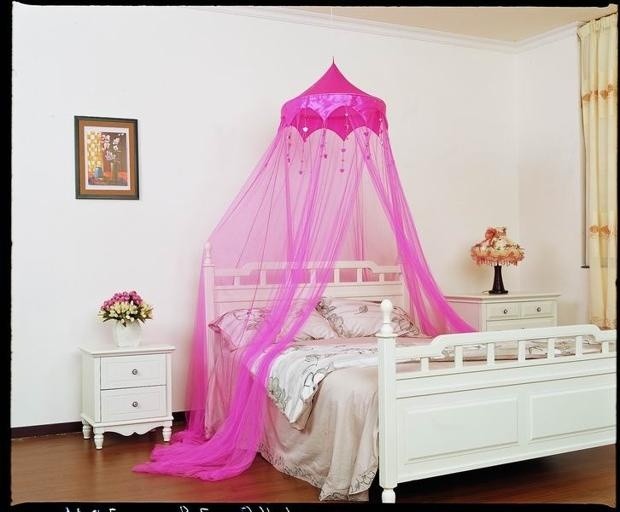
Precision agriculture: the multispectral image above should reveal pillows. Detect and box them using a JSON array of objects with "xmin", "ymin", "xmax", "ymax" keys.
[
  {"xmin": 314, "ymin": 294, "xmax": 424, "ymax": 338},
  {"xmin": 206, "ymin": 299, "xmax": 339, "ymax": 354}
]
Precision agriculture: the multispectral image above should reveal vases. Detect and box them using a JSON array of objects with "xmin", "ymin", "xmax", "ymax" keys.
[
  {"xmin": 484, "ymin": 264, "xmax": 513, "ymax": 297},
  {"xmin": 111, "ymin": 316, "xmax": 147, "ymax": 349}
]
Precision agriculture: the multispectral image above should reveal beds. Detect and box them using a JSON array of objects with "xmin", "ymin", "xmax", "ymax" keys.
[{"xmin": 201, "ymin": 250, "xmax": 620, "ymax": 510}]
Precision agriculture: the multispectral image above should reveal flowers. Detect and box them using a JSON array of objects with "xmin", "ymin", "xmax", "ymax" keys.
[
  {"xmin": 95, "ymin": 288, "xmax": 156, "ymax": 329},
  {"xmin": 468, "ymin": 223, "xmax": 527, "ymax": 270}
]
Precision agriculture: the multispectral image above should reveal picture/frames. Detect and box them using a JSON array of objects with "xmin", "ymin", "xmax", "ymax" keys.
[{"xmin": 72, "ymin": 113, "xmax": 144, "ymax": 204}]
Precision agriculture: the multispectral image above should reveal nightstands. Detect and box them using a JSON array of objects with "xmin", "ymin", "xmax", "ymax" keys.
[
  {"xmin": 78, "ymin": 340, "xmax": 177, "ymax": 452},
  {"xmin": 437, "ymin": 289, "xmax": 563, "ymax": 334}
]
